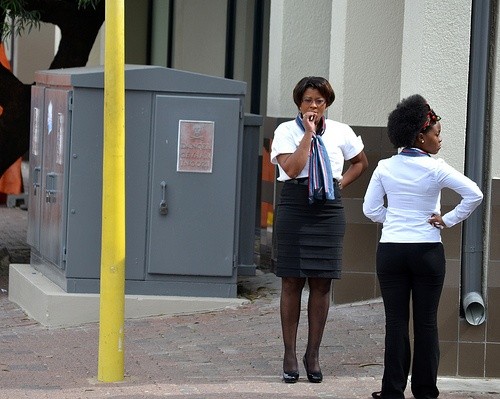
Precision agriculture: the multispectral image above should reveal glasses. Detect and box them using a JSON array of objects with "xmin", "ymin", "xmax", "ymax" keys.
[{"xmin": 303, "ymin": 97, "xmax": 326, "ymax": 106}]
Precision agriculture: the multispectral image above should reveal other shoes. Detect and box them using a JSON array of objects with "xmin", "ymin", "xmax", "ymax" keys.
[{"xmin": 371, "ymin": 391, "xmax": 383, "ymax": 399}]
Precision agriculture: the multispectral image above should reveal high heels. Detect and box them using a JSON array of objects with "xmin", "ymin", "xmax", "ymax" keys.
[
  {"xmin": 283, "ymin": 357, "xmax": 299, "ymax": 383},
  {"xmin": 303, "ymin": 353, "xmax": 322, "ymax": 382}
]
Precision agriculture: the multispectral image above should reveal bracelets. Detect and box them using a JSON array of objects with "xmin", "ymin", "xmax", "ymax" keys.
[{"xmin": 338, "ymin": 182, "xmax": 342, "ymax": 189}]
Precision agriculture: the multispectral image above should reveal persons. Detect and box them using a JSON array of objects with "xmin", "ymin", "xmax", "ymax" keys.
[
  {"xmin": 363, "ymin": 95, "xmax": 484, "ymax": 399},
  {"xmin": 271, "ymin": 76, "xmax": 369, "ymax": 382}
]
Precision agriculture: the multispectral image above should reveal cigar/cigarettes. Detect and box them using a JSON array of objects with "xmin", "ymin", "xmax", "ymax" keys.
[{"xmin": 310, "ymin": 116, "xmax": 313, "ymax": 120}]
